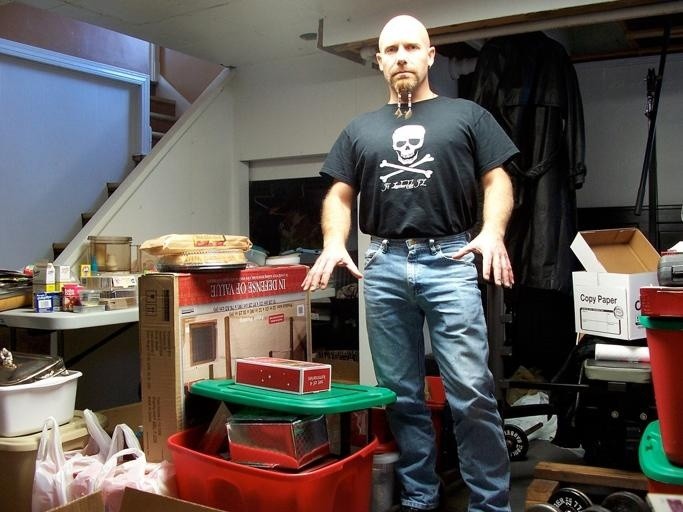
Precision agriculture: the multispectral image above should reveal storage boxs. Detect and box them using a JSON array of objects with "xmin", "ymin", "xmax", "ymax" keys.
[{"xmin": 559, "ymin": 223, "xmax": 682, "ymax": 511}]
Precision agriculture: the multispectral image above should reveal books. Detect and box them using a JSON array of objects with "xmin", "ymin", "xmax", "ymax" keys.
[{"xmin": 264, "ymin": 252, "xmax": 319, "ymax": 266}]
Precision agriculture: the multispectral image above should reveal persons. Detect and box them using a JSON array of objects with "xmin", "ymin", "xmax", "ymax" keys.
[{"xmin": 299, "ymin": 14, "xmax": 521, "ymax": 511}]
